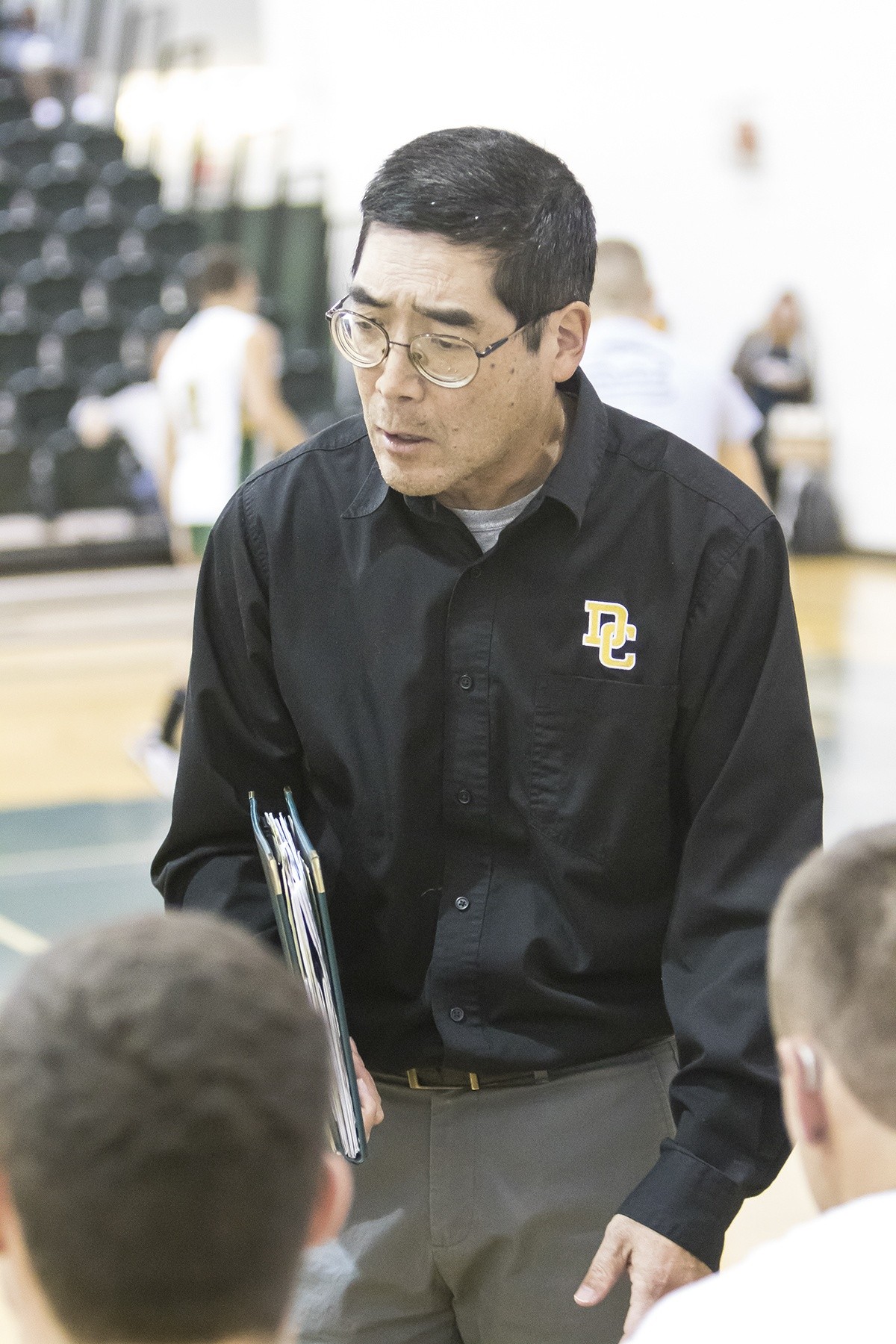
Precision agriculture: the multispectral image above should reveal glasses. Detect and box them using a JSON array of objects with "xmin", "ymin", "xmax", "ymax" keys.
[{"xmin": 324, "ymin": 286, "xmax": 551, "ymax": 389}]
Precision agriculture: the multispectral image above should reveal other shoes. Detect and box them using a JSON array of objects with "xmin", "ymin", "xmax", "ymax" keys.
[{"xmin": 139, "ymin": 737, "xmax": 180, "ymax": 793}]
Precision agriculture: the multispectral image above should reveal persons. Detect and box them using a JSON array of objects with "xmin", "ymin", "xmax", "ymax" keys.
[
  {"xmin": 31, "ymin": 240, "xmax": 312, "ymax": 565},
  {"xmin": 579, "ymin": 240, "xmax": 770, "ymax": 510},
  {"xmin": 732, "ymin": 290, "xmax": 834, "ymax": 552},
  {"xmin": 148, "ymin": 125, "xmax": 824, "ymax": 1344},
  {"xmin": 623, "ymin": 820, "xmax": 896, "ymax": 1344},
  {"xmin": 0, "ymin": 910, "xmax": 354, "ymax": 1344}
]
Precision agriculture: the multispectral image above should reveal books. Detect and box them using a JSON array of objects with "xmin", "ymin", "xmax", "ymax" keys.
[{"xmin": 246, "ymin": 786, "xmax": 367, "ymax": 1163}]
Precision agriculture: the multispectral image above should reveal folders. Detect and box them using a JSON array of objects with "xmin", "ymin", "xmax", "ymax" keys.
[{"xmin": 246, "ymin": 785, "xmax": 366, "ymax": 1164}]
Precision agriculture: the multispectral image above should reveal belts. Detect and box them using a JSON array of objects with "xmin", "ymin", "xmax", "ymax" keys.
[{"xmin": 366, "ymin": 1059, "xmax": 558, "ymax": 1090}]
